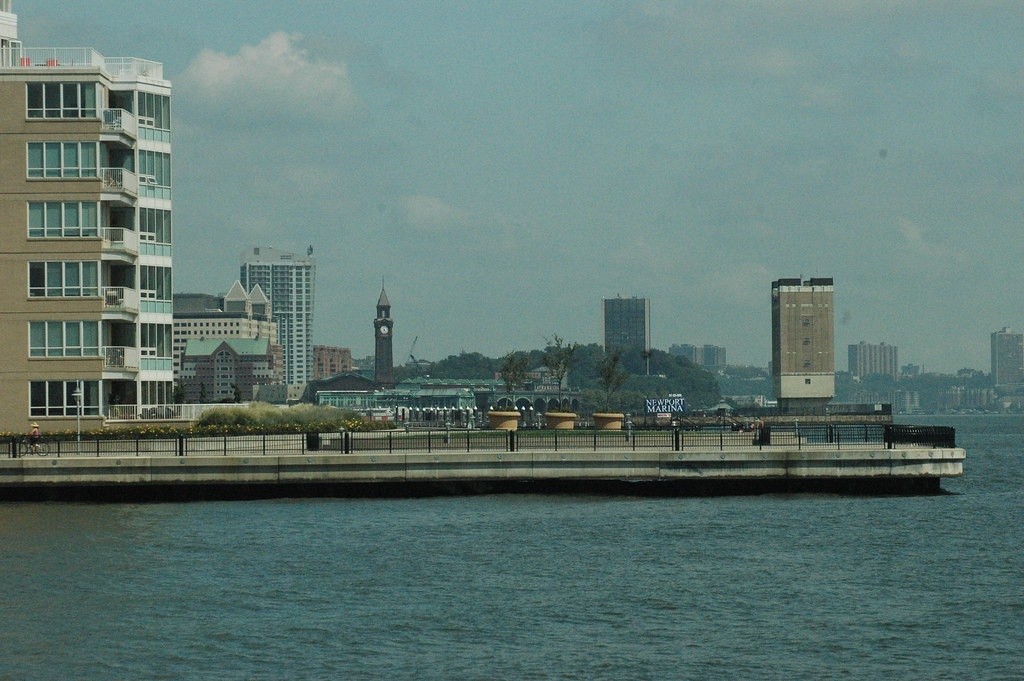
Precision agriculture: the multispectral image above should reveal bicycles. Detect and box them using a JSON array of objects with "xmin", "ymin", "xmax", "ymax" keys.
[{"xmin": 15, "ymin": 436, "xmax": 50, "ymax": 456}]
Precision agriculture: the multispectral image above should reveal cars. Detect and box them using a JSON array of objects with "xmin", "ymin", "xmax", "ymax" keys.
[{"xmin": 141, "ymin": 406, "xmax": 174, "ymax": 419}]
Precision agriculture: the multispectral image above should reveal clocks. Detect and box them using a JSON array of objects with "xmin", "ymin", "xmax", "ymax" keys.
[{"xmin": 380, "ymin": 325, "xmax": 389, "ymax": 334}]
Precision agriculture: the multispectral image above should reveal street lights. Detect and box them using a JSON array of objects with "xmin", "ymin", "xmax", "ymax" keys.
[
  {"xmin": 396, "ymin": 405, "xmax": 478, "ymax": 427},
  {"xmin": 522, "ymin": 406, "xmax": 534, "ymax": 426},
  {"xmin": 72, "ymin": 388, "xmax": 83, "ymax": 453}
]
